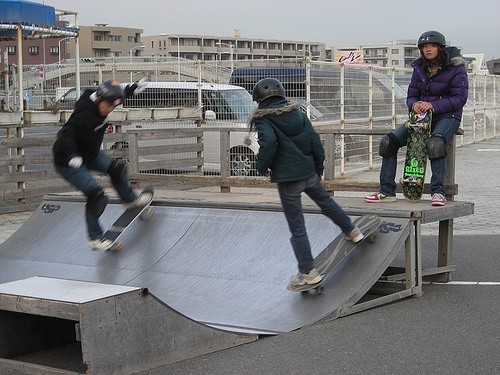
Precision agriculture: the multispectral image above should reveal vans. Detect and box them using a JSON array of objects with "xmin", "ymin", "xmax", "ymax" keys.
[
  {"xmin": 100, "ymin": 81, "xmax": 272, "ymax": 176},
  {"xmin": 47, "ymin": 85, "xmax": 99, "ymax": 110},
  {"xmin": 229, "ymin": 66, "xmax": 409, "ymax": 161}
]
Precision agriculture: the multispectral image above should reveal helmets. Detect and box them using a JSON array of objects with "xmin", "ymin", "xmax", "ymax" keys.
[
  {"xmin": 95, "ymin": 79, "xmax": 124, "ymax": 106},
  {"xmin": 418, "ymin": 30, "xmax": 447, "ymax": 46},
  {"xmin": 252, "ymin": 77, "xmax": 286, "ymax": 104}
]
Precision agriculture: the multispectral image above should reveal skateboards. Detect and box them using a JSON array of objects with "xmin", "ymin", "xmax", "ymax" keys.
[
  {"xmin": 91, "ymin": 189, "xmax": 154, "ymax": 251},
  {"xmin": 399, "ymin": 102, "xmax": 432, "ymax": 202},
  {"xmin": 286, "ymin": 213, "xmax": 381, "ymax": 294}
]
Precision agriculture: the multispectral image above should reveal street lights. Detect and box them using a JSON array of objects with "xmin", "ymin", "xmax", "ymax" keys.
[
  {"xmin": 59, "ymin": 37, "xmax": 75, "ymax": 88},
  {"xmin": 161, "ymin": 34, "xmax": 180, "ymax": 82},
  {"xmin": 298, "ymin": 50, "xmax": 311, "ymax": 60},
  {"xmin": 216, "ymin": 43, "xmax": 234, "ymax": 73},
  {"xmin": 130, "ymin": 46, "xmax": 146, "ymax": 83}
]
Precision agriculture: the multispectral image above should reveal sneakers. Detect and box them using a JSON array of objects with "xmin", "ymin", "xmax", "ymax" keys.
[
  {"xmin": 343, "ymin": 226, "xmax": 364, "ymax": 244},
  {"xmin": 289, "ymin": 268, "xmax": 322, "ymax": 286},
  {"xmin": 364, "ymin": 190, "xmax": 397, "ymax": 203},
  {"xmin": 430, "ymin": 192, "xmax": 448, "ymax": 205},
  {"xmin": 124, "ymin": 195, "xmax": 153, "ymax": 210},
  {"xmin": 87, "ymin": 237, "xmax": 114, "ymax": 252}
]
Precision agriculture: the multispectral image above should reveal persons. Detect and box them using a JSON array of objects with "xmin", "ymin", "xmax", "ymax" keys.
[
  {"xmin": 364, "ymin": 31, "xmax": 469, "ymax": 206},
  {"xmin": 248, "ymin": 78, "xmax": 364, "ymax": 286},
  {"xmin": 53, "ymin": 76, "xmax": 152, "ymax": 252}
]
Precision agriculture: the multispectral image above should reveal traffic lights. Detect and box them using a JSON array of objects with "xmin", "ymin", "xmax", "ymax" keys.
[{"xmin": 81, "ymin": 58, "xmax": 91, "ymax": 62}]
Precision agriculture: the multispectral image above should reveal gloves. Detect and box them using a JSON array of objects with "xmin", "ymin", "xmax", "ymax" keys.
[
  {"xmin": 134, "ymin": 77, "xmax": 149, "ymax": 96},
  {"xmin": 67, "ymin": 155, "xmax": 84, "ymax": 170}
]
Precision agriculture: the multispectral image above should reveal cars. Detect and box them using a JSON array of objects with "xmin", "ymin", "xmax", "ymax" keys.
[
  {"xmin": 400, "ymin": 85, "xmax": 495, "ymax": 131},
  {"xmin": 286, "ymin": 97, "xmax": 355, "ymax": 166}
]
